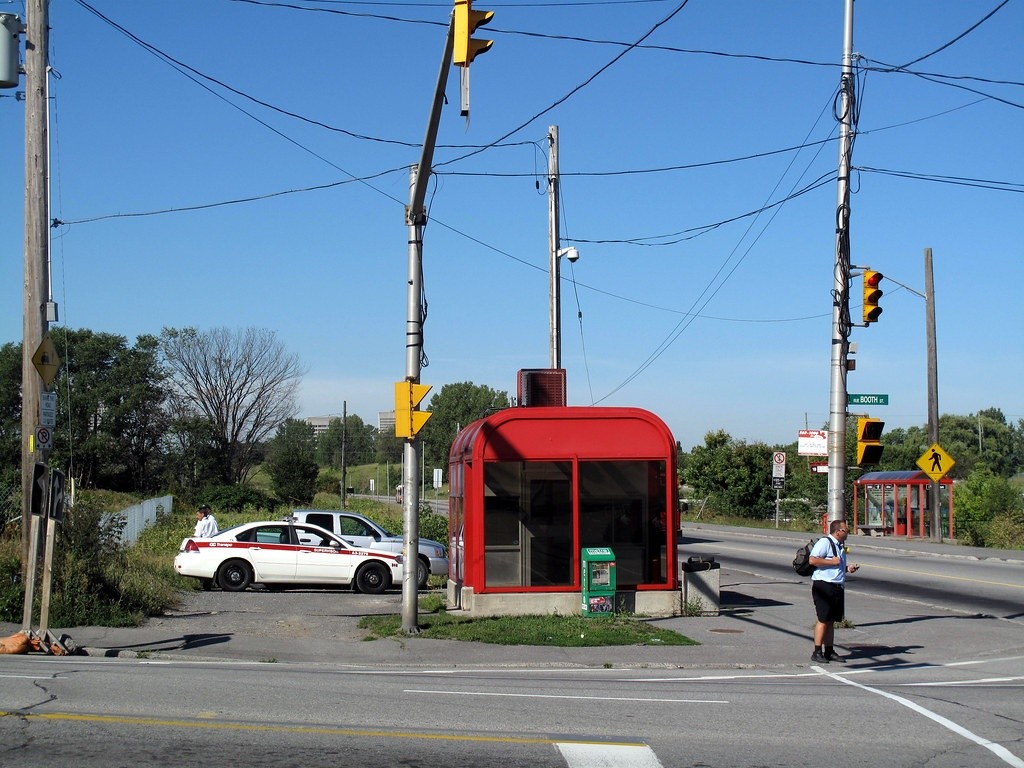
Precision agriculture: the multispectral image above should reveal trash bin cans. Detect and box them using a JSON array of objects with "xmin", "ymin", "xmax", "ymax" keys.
[{"xmin": 897, "ymin": 517, "xmax": 907, "ymax": 535}]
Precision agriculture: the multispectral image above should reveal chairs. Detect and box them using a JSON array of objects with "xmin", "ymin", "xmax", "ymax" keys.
[{"xmin": 279, "ymin": 533, "xmax": 289, "ymax": 543}]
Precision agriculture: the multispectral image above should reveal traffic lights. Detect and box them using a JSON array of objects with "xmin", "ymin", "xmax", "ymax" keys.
[
  {"xmin": 395, "ymin": 383, "xmax": 435, "ymax": 442},
  {"xmin": 857, "ymin": 417, "xmax": 885, "ymax": 466},
  {"xmin": 454, "ymin": 1, "xmax": 496, "ymax": 69},
  {"xmin": 862, "ymin": 269, "xmax": 883, "ymax": 324}
]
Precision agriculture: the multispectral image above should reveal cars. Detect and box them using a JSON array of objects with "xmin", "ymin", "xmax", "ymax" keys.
[{"xmin": 173, "ymin": 512, "xmax": 404, "ymax": 595}]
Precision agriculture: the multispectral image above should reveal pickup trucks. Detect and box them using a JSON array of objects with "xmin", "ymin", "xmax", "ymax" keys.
[{"xmin": 253, "ymin": 511, "xmax": 451, "ymax": 590}]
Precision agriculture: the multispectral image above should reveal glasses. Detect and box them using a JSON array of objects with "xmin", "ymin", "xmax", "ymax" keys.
[{"xmin": 838, "ymin": 528, "xmax": 848, "ymax": 534}]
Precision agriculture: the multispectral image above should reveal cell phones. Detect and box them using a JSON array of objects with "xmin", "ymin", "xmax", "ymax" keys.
[{"xmin": 854, "ymin": 566, "xmax": 860, "ymax": 569}]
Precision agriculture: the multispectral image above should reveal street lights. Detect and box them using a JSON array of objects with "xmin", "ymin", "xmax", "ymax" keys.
[
  {"xmin": 849, "ymin": 247, "xmax": 944, "ymax": 543},
  {"xmin": 547, "ymin": 246, "xmax": 581, "ymax": 368}
]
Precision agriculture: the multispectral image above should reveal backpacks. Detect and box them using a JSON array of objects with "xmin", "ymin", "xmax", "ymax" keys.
[{"xmin": 792, "ymin": 536, "xmax": 837, "ymax": 577}]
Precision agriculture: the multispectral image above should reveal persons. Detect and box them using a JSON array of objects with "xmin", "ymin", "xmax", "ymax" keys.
[
  {"xmin": 808, "ymin": 519, "xmax": 857, "ymax": 663},
  {"xmin": 192, "ymin": 502, "xmax": 219, "ymax": 591}
]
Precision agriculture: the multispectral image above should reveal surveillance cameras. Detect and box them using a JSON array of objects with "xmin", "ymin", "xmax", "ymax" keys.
[{"xmin": 567, "ymin": 250, "xmax": 579, "ymax": 262}]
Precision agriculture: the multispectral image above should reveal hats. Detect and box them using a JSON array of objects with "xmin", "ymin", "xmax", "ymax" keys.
[{"xmin": 198, "ymin": 504, "xmax": 211, "ymax": 510}]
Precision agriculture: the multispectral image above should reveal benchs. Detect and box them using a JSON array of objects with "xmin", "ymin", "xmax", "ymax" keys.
[{"xmin": 856, "ymin": 525, "xmax": 885, "ymax": 536}]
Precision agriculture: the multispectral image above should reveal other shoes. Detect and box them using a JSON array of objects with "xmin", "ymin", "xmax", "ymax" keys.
[
  {"xmin": 823, "ymin": 650, "xmax": 845, "ymax": 663},
  {"xmin": 811, "ymin": 651, "xmax": 829, "ymax": 663}
]
